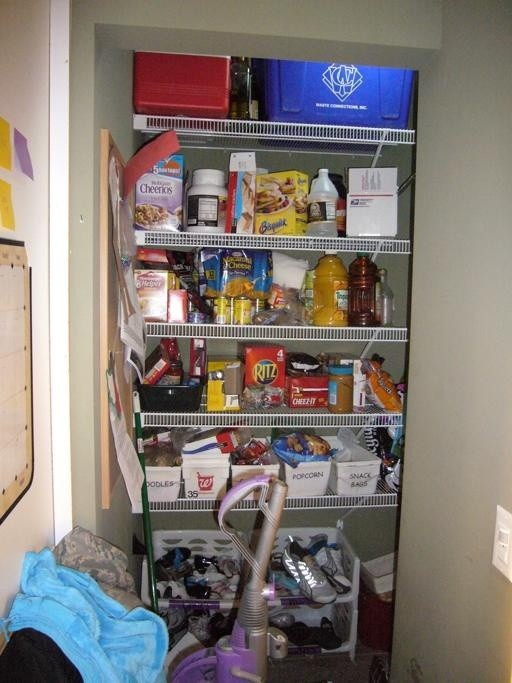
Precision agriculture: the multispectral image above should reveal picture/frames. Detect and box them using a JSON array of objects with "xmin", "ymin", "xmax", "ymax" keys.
[{"xmin": 96, "ymin": 129, "xmax": 132, "ymax": 510}]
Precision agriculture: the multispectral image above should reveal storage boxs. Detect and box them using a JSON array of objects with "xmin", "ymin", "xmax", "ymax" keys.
[
  {"xmin": 254, "ymin": 58, "xmax": 414, "ymax": 128},
  {"xmin": 362, "ymin": 551, "xmax": 396, "ymax": 594},
  {"xmin": 139, "ymin": 433, "xmax": 385, "ymax": 504},
  {"xmin": 133, "ymin": 50, "xmax": 231, "ymax": 118}
]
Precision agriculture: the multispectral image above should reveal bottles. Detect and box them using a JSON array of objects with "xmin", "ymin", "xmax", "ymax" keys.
[
  {"xmin": 181, "ymin": 167, "xmax": 229, "ymax": 234},
  {"xmin": 230, "ymin": 56, "xmax": 253, "ymax": 120},
  {"xmin": 307, "ymin": 165, "xmax": 347, "ymax": 241},
  {"xmin": 300, "ymin": 248, "xmax": 396, "ymax": 327}
]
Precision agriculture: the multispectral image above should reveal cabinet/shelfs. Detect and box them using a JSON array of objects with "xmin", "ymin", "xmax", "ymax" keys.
[
  {"xmin": 120, "ymin": 105, "xmax": 417, "ymax": 660},
  {"xmin": 251, "ymin": 525, "xmax": 361, "ymax": 661},
  {"xmin": 139, "ymin": 528, "xmax": 250, "ymax": 660}
]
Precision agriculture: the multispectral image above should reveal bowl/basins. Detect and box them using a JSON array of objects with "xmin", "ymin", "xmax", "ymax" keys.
[{"xmin": 134, "ymin": 376, "xmax": 207, "ymax": 412}]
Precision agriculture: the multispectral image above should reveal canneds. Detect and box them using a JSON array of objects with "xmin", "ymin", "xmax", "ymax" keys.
[
  {"xmin": 166, "ymin": 360, "xmax": 183, "ymax": 386},
  {"xmin": 206, "ymin": 296, "xmax": 266, "ymax": 325},
  {"xmin": 328, "ymin": 366, "xmax": 353, "ymax": 415}
]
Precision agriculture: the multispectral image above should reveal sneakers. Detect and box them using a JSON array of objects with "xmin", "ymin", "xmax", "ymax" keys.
[
  {"xmin": 281, "ymin": 542, "xmax": 352, "ymax": 605},
  {"xmin": 320, "ymin": 617, "xmax": 342, "ymax": 651}
]
